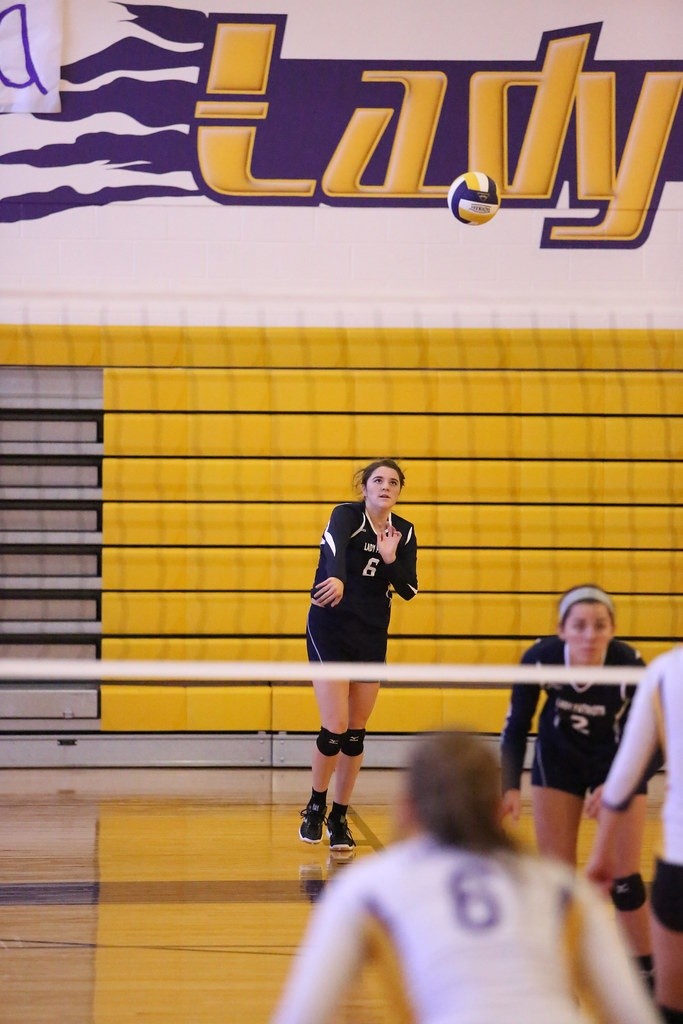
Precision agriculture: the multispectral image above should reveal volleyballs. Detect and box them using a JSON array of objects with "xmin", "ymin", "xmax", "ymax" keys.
[{"xmin": 447, "ymin": 171, "xmax": 502, "ymax": 226}]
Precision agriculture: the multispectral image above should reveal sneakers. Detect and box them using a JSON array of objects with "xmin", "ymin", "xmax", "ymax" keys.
[
  {"xmin": 299, "ymin": 800, "xmax": 329, "ymax": 844},
  {"xmin": 326, "ymin": 811, "xmax": 356, "ymax": 851}
]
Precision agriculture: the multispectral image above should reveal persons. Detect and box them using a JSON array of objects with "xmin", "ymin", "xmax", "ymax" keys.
[
  {"xmin": 499, "ymin": 583, "xmax": 655, "ymax": 999},
  {"xmin": 268, "ymin": 729, "xmax": 667, "ymax": 1024},
  {"xmin": 299, "ymin": 456, "xmax": 417, "ymax": 852},
  {"xmin": 584, "ymin": 646, "xmax": 683, "ymax": 1024}
]
